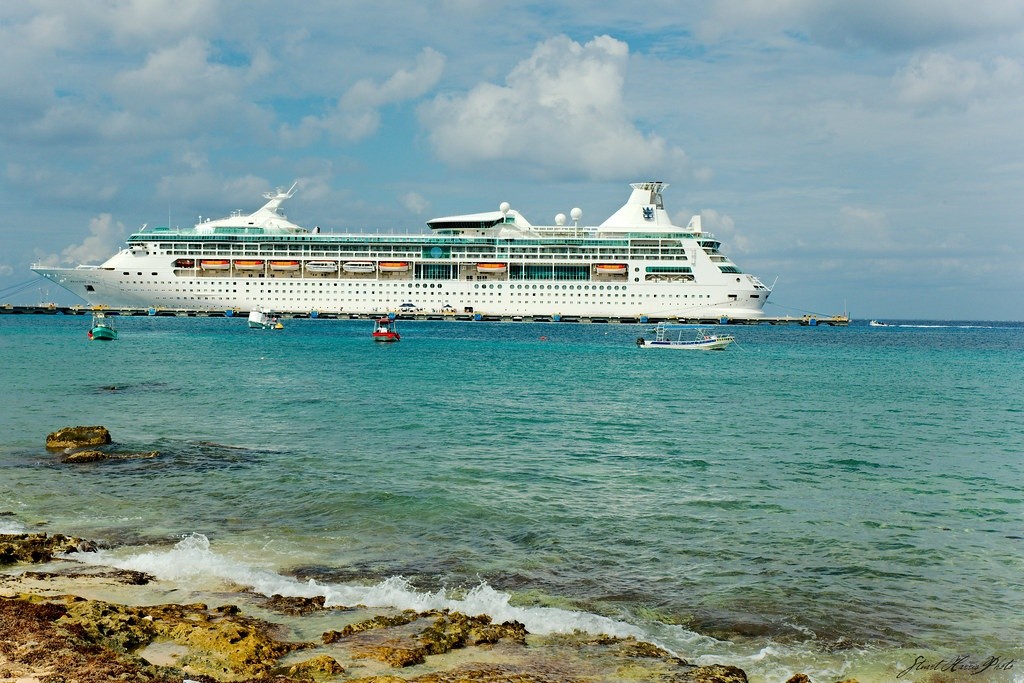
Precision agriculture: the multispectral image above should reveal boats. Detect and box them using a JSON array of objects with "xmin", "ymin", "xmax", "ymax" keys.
[
  {"xmin": 87, "ymin": 310, "xmax": 118, "ymax": 341},
  {"xmin": 372, "ymin": 315, "xmax": 401, "ymax": 343},
  {"xmin": 636, "ymin": 321, "xmax": 735, "ymax": 352},
  {"xmin": 244, "ymin": 306, "xmax": 285, "ymax": 332}
]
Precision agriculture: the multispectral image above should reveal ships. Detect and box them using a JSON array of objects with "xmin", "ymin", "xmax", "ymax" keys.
[{"xmin": 28, "ymin": 176, "xmax": 774, "ymax": 316}]
